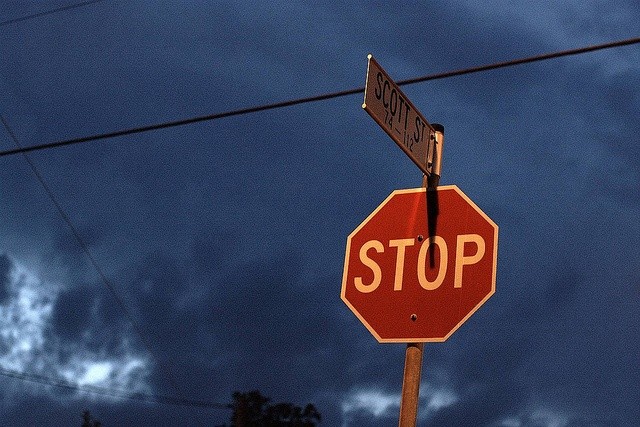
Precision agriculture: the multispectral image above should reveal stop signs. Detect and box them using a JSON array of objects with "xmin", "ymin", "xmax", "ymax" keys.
[{"xmin": 339, "ymin": 185, "xmax": 498, "ymax": 342}]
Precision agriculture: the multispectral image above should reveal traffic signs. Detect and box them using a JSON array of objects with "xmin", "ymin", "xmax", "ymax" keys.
[{"xmin": 363, "ymin": 54, "xmax": 432, "ymax": 177}]
